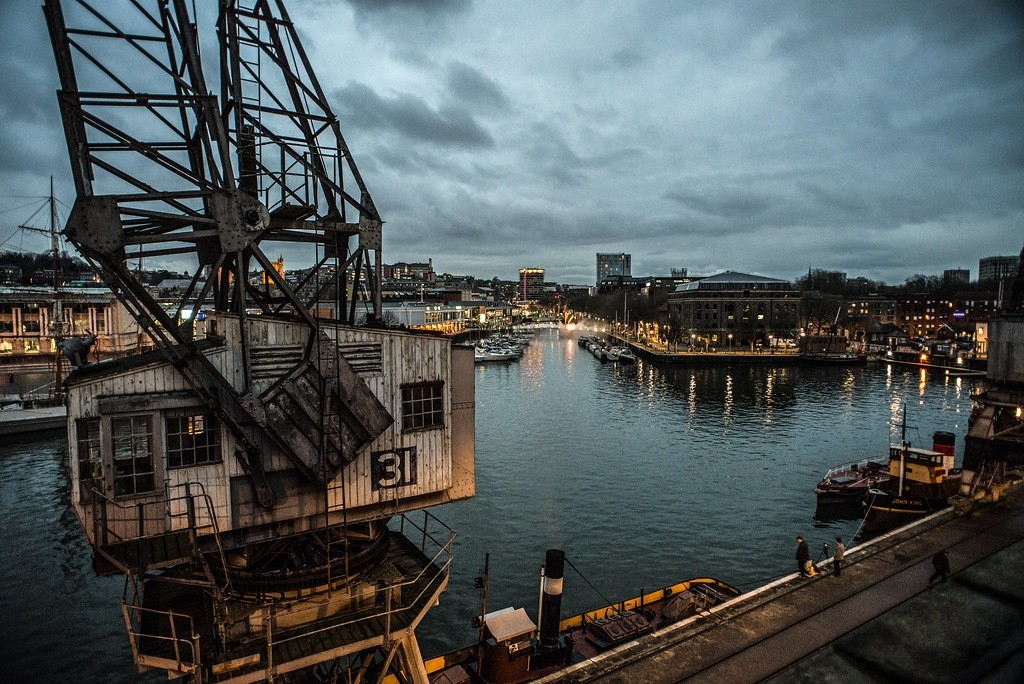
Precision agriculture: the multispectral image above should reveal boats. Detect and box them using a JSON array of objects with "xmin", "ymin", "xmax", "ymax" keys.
[
  {"xmin": 813, "ymin": 454, "xmax": 892, "ymax": 509},
  {"xmin": 577, "ymin": 332, "xmax": 635, "ymax": 368},
  {"xmin": 381, "ymin": 572, "xmax": 743, "ymax": 684},
  {"xmin": 469, "ymin": 327, "xmax": 535, "ymax": 361}
]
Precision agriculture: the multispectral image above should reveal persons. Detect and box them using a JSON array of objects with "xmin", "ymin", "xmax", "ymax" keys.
[
  {"xmin": 833, "ymin": 536, "xmax": 845, "ymax": 577},
  {"xmin": 795, "ymin": 536, "xmax": 811, "ymax": 577}
]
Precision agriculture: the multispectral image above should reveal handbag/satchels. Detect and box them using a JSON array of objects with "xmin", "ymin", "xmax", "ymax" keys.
[
  {"xmin": 804, "ymin": 560, "xmax": 812, "ymax": 571},
  {"xmin": 812, "ymin": 564, "xmax": 819, "ymax": 572}
]
